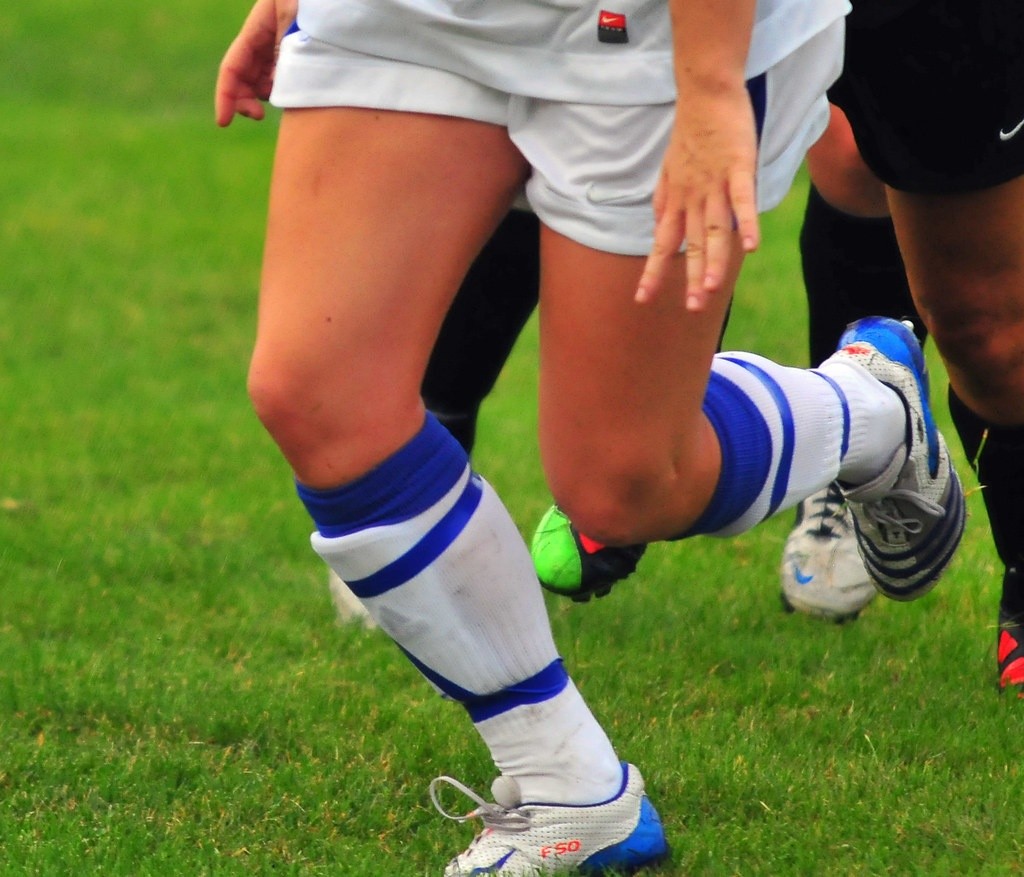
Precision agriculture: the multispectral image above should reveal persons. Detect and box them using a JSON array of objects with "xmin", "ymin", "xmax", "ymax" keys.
[
  {"xmin": 417, "ymin": 0, "xmax": 1024, "ymax": 690},
  {"xmin": 216, "ymin": 0, "xmax": 969, "ymax": 877}
]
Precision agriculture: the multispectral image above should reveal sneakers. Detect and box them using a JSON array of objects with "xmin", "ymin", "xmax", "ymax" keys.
[
  {"xmin": 831, "ymin": 318, "xmax": 967, "ymax": 604},
  {"xmin": 429, "ymin": 762, "xmax": 671, "ymax": 877},
  {"xmin": 997, "ymin": 606, "xmax": 1024, "ymax": 699},
  {"xmin": 327, "ymin": 565, "xmax": 378, "ymax": 628},
  {"xmin": 778, "ymin": 481, "xmax": 879, "ymax": 621}
]
